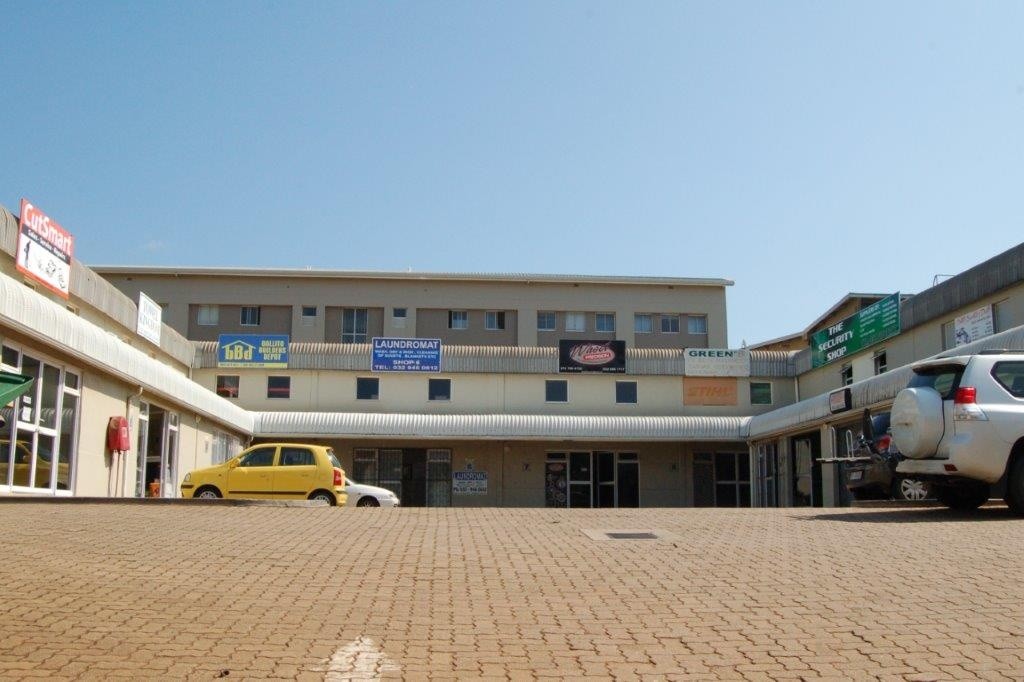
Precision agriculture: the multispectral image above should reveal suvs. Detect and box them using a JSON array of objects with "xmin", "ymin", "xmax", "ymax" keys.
[
  {"xmin": 845, "ymin": 411, "xmax": 929, "ymax": 501},
  {"xmin": 889, "ymin": 348, "xmax": 1024, "ymax": 513}
]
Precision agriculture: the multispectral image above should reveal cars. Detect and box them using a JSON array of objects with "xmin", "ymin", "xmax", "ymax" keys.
[
  {"xmin": 344, "ymin": 478, "xmax": 400, "ymax": 508},
  {"xmin": 181, "ymin": 442, "xmax": 347, "ymax": 508}
]
